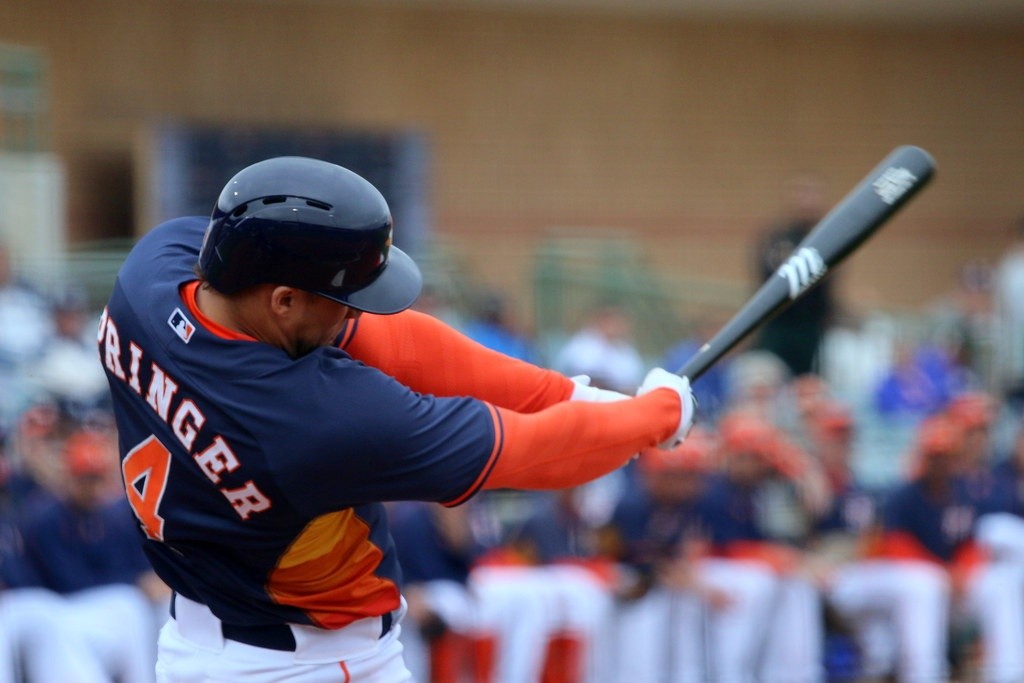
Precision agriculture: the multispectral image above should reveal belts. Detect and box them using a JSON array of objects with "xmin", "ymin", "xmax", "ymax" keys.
[{"xmin": 169, "ymin": 590, "xmax": 392, "ymax": 652}]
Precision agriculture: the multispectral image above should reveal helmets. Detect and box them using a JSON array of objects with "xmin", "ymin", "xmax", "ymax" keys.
[{"xmin": 198, "ymin": 156, "xmax": 422, "ymax": 315}]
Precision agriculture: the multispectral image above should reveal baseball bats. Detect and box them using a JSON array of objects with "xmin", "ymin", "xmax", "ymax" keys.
[{"xmin": 680, "ymin": 141, "xmax": 943, "ymax": 383}]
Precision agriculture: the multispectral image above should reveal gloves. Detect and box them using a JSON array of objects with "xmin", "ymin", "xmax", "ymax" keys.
[
  {"xmin": 569, "ymin": 375, "xmax": 642, "ymax": 467},
  {"xmin": 636, "ymin": 366, "xmax": 699, "ymax": 451}
]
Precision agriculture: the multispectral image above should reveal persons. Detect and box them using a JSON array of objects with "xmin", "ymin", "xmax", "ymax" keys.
[
  {"xmin": 0, "ymin": 176, "xmax": 1024, "ymax": 682},
  {"xmin": 99, "ymin": 154, "xmax": 693, "ymax": 682}
]
